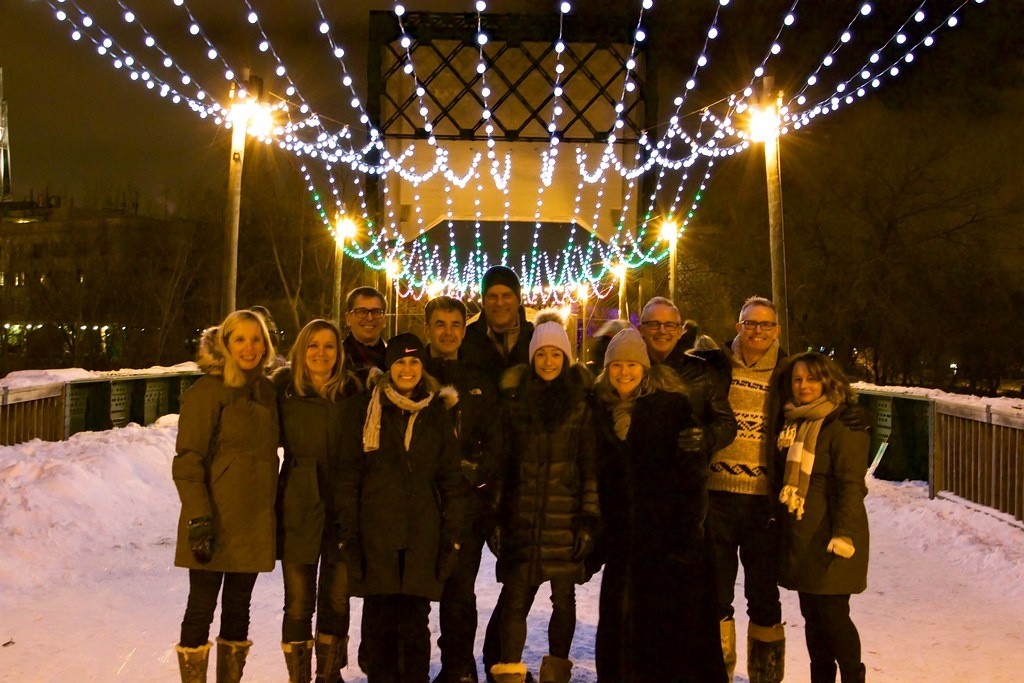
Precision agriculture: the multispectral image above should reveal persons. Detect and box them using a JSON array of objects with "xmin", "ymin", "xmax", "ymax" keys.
[
  {"xmin": 275, "ymin": 319, "xmax": 365, "ymax": 683},
  {"xmin": 171, "ymin": 311, "xmax": 279, "ymax": 683},
  {"xmin": 590, "ymin": 327, "xmax": 733, "ymax": 683},
  {"xmin": 639, "ymin": 297, "xmax": 740, "ymax": 462},
  {"xmin": 459, "ymin": 264, "xmax": 534, "ymax": 683},
  {"xmin": 704, "ymin": 296, "xmax": 872, "ymax": 683},
  {"xmin": 474, "ymin": 310, "xmax": 601, "ymax": 683},
  {"xmin": 349, "ymin": 335, "xmax": 467, "ymax": 683},
  {"xmin": 413, "ymin": 297, "xmax": 504, "ymax": 683},
  {"xmin": 336, "ymin": 289, "xmax": 386, "ymax": 397},
  {"xmin": 776, "ymin": 352, "xmax": 871, "ymax": 683}
]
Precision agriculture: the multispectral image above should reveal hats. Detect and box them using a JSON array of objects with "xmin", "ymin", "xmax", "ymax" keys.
[
  {"xmin": 481, "ymin": 265, "xmax": 521, "ymax": 304},
  {"xmin": 604, "ymin": 327, "xmax": 651, "ymax": 370},
  {"xmin": 529, "ymin": 308, "xmax": 572, "ymax": 367},
  {"xmin": 386, "ymin": 333, "xmax": 426, "ymax": 371}
]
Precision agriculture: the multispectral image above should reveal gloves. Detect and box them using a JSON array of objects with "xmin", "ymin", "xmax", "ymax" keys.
[
  {"xmin": 188, "ymin": 516, "xmax": 215, "ymax": 564},
  {"xmin": 437, "ymin": 537, "xmax": 464, "ymax": 582},
  {"xmin": 334, "ymin": 522, "xmax": 358, "ymax": 561},
  {"xmin": 571, "ymin": 520, "xmax": 594, "ymax": 563},
  {"xmin": 841, "ymin": 403, "xmax": 874, "ymax": 431},
  {"xmin": 677, "ymin": 412, "xmax": 714, "ymax": 452},
  {"xmin": 486, "ymin": 520, "xmax": 501, "ymax": 559},
  {"xmin": 826, "ymin": 535, "xmax": 855, "ymax": 558}
]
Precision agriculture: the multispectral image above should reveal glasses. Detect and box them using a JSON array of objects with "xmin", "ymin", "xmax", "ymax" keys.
[
  {"xmin": 740, "ymin": 320, "xmax": 777, "ymax": 331},
  {"xmin": 350, "ymin": 307, "xmax": 385, "ymax": 319},
  {"xmin": 641, "ymin": 320, "xmax": 682, "ymax": 331}
]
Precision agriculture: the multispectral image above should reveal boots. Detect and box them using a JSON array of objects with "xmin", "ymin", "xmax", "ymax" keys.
[
  {"xmin": 175, "ymin": 640, "xmax": 213, "ymax": 683},
  {"xmin": 839, "ymin": 662, "xmax": 866, "ymax": 683},
  {"xmin": 491, "ymin": 662, "xmax": 528, "ymax": 683},
  {"xmin": 215, "ymin": 635, "xmax": 254, "ymax": 683},
  {"xmin": 538, "ymin": 654, "xmax": 573, "ymax": 683},
  {"xmin": 811, "ymin": 662, "xmax": 837, "ymax": 683},
  {"xmin": 314, "ymin": 628, "xmax": 350, "ymax": 683},
  {"xmin": 719, "ymin": 616, "xmax": 737, "ymax": 683},
  {"xmin": 748, "ymin": 620, "xmax": 786, "ymax": 683},
  {"xmin": 282, "ymin": 638, "xmax": 314, "ymax": 683}
]
